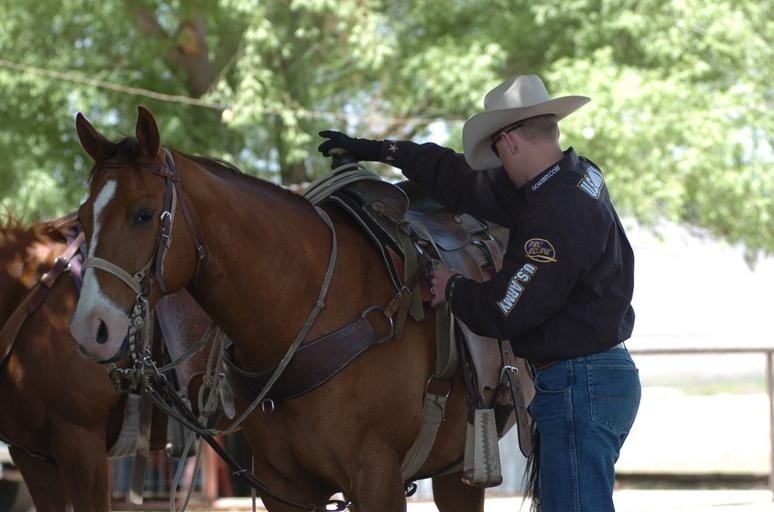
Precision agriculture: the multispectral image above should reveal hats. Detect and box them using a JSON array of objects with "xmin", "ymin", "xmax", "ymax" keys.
[{"xmin": 461, "ymin": 72, "xmax": 591, "ymax": 169}]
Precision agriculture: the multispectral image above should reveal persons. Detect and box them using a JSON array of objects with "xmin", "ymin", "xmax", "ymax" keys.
[{"xmin": 318, "ymin": 74, "xmax": 642, "ymax": 512}]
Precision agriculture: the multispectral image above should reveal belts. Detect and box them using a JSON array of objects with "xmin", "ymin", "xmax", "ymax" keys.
[{"xmin": 523, "ymin": 341, "xmax": 627, "ymax": 372}]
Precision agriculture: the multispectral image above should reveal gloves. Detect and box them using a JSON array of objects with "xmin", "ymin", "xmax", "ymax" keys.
[{"xmin": 319, "ymin": 130, "xmax": 383, "ymax": 162}]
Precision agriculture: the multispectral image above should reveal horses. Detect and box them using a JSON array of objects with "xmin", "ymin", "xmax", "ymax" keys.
[
  {"xmin": 69, "ymin": 105, "xmax": 540, "ymax": 512},
  {"xmin": 0, "ymin": 203, "xmax": 233, "ymax": 512}
]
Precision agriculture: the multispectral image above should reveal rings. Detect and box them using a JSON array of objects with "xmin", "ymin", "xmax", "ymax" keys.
[{"xmin": 430, "ymin": 286, "xmax": 436, "ymax": 295}]
